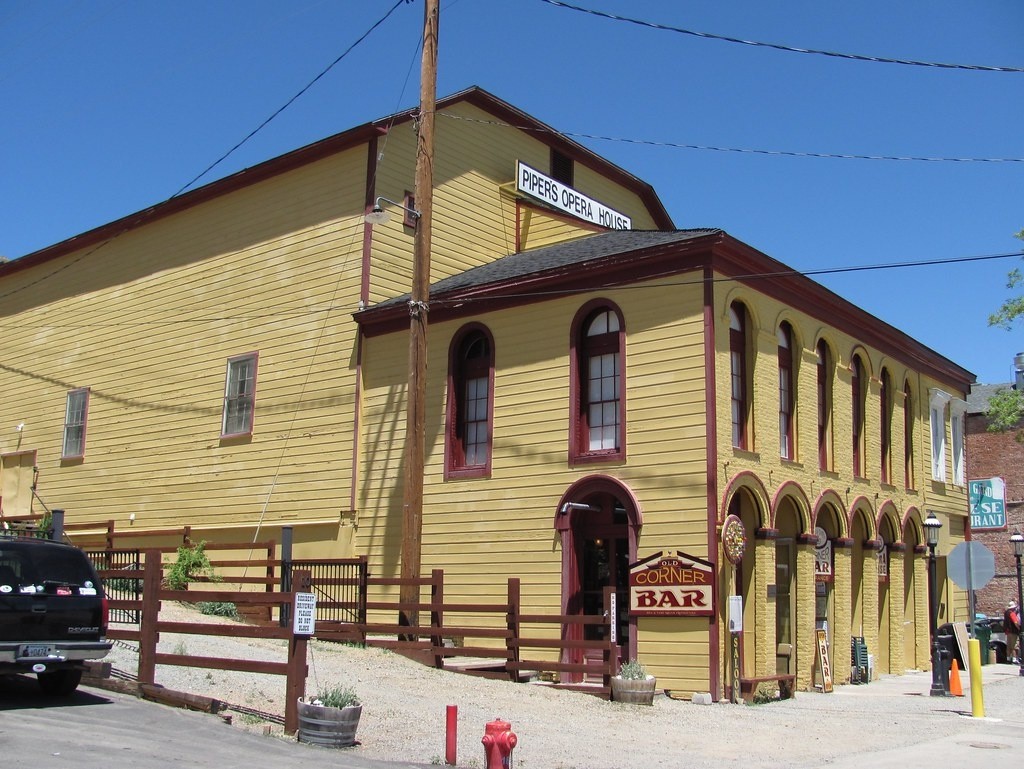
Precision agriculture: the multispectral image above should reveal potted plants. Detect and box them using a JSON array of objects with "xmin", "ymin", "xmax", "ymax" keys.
[
  {"xmin": 296, "ymin": 684, "xmax": 364, "ymax": 749},
  {"xmin": 609, "ymin": 659, "xmax": 657, "ymax": 705}
]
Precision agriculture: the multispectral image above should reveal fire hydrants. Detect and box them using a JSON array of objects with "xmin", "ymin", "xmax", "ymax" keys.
[{"xmin": 481, "ymin": 717, "xmax": 518, "ymax": 769}]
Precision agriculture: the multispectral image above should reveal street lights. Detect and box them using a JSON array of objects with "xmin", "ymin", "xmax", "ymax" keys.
[
  {"xmin": 1009, "ymin": 528, "xmax": 1024, "ymax": 676},
  {"xmin": 922, "ymin": 509, "xmax": 950, "ymax": 697}
]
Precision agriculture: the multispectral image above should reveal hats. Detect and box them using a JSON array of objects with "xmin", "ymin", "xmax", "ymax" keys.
[{"xmin": 1007, "ymin": 602, "xmax": 1017, "ymax": 610}]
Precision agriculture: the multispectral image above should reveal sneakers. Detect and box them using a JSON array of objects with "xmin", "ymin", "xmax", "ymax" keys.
[{"xmin": 1006, "ymin": 661, "xmax": 1021, "ymax": 665}]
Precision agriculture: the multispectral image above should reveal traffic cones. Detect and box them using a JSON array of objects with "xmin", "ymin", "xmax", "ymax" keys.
[{"xmin": 947, "ymin": 658, "xmax": 966, "ymax": 697}]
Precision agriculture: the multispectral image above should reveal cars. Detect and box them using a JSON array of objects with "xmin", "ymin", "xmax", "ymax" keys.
[{"xmin": 933, "ymin": 615, "xmax": 1021, "ymax": 664}]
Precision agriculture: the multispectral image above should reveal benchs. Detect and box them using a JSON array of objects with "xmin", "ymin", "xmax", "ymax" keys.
[{"xmin": 740, "ymin": 671, "xmax": 796, "ymax": 703}]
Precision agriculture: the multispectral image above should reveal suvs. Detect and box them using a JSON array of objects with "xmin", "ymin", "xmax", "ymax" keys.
[{"xmin": 0, "ymin": 523, "xmax": 115, "ymax": 698}]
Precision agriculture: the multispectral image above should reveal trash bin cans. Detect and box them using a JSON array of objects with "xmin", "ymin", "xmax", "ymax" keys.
[{"xmin": 973, "ymin": 618, "xmax": 991, "ymax": 666}]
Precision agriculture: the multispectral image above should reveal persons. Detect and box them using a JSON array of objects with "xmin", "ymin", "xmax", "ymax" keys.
[{"xmin": 1002, "ymin": 601, "xmax": 1020, "ymax": 664}]
